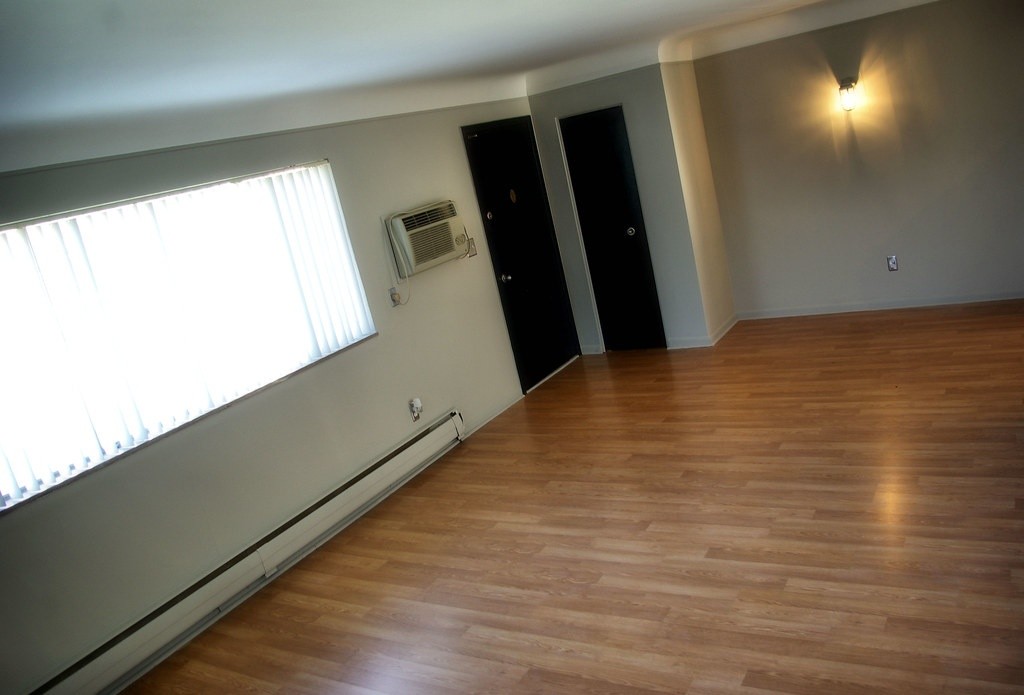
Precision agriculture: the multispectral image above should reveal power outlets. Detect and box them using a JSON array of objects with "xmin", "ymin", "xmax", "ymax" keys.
[
  {"xmin": 388, "ymin": 287, "xmax": 400, "ymax": 307},
  {"xmin": 887, "ymin": 255, "xmax": 898, "ymax": 272},
  {"xmin": 409, "ymin": 406, "xmax": 420, "ymax": 422}
]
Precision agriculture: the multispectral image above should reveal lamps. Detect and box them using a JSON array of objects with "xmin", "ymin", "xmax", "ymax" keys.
[{"xmin": 839, "ymin": 78, "xmax": 857, "ymax": 111}]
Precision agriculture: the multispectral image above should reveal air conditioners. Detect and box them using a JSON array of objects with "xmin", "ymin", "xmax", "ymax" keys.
[{"xmin": 387, "ymin": 199, "xmax": 468, "ymax": 278}]
